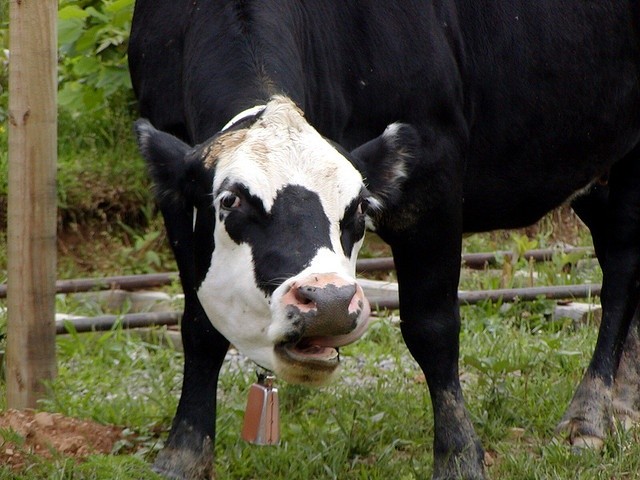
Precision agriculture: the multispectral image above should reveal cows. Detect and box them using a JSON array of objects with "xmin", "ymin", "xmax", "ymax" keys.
[{"xmin": 129, "ymin": 0, "xmax": 640, "ymax": 480}]
